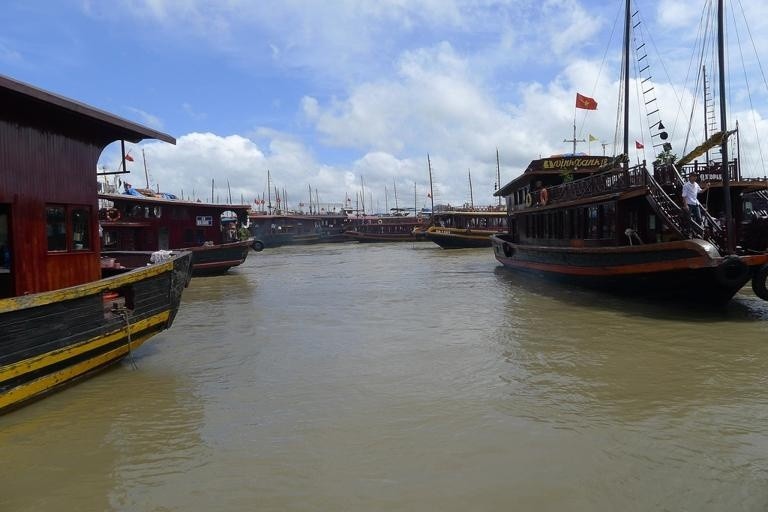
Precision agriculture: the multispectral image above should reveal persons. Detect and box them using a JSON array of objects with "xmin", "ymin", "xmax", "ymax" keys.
[{"xmin": 681, "ymin": 171, "xmax": 711, "ymax": 226}]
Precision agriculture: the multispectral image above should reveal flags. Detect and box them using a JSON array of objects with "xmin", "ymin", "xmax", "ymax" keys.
[
  {"xmin": 635, "ymin": 142, "xmax": 645, "ymax": 150},
  {"xmin": 576, "ymin": 93, "xmax": 599, "ymax": 112},
  {"xmin": 589, "ymin": 134, "xmax": 599, "ymax": 142},
  {"xmin": 125, "ymin": 154, "xmax": 135, "ymax": 162}
]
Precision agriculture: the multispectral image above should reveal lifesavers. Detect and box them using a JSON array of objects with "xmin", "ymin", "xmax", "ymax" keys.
[
  {"xmin": 540, "ymin": 189, "xmax": 547, "ymax": 205},
  {"xmin": 526, "ymin": 194, "xmax": 532, "ymax": 207},
  {"xmin": 107, "ymin": 208, "xmax": 120, "ymax": 221},
  {"xmin": 378, "ymin": 220, "xmax": 382, "ymax": 224},
  {"xmin": 497, "ymin": 227, "xmax": 503, "ymax": 232},
  {"xmin": 466, "ymin": 229, "xmax": 472, "ymax": 234},
  {"xmin": 417, "ymin": 218, "xmax": 423, "ymax": 223}
]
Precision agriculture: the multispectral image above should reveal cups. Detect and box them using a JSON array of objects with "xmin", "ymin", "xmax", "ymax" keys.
[{"xmin": 75, "ymin": 244, "xmax": 84, "ymax": 250}]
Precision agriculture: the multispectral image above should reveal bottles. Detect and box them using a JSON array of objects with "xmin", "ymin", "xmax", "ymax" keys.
[
  {"xmin": 4, "ymin": 247, "xmax": 11, "ymax": 265},
  {"xmin": 114, "ymin": 257, "xmax": 120, "ymax": 274}
]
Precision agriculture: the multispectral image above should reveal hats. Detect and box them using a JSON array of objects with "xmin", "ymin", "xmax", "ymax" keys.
[{"xmin": 689, "ymin": 172, "xmax": 700, "ymax": 176}]
